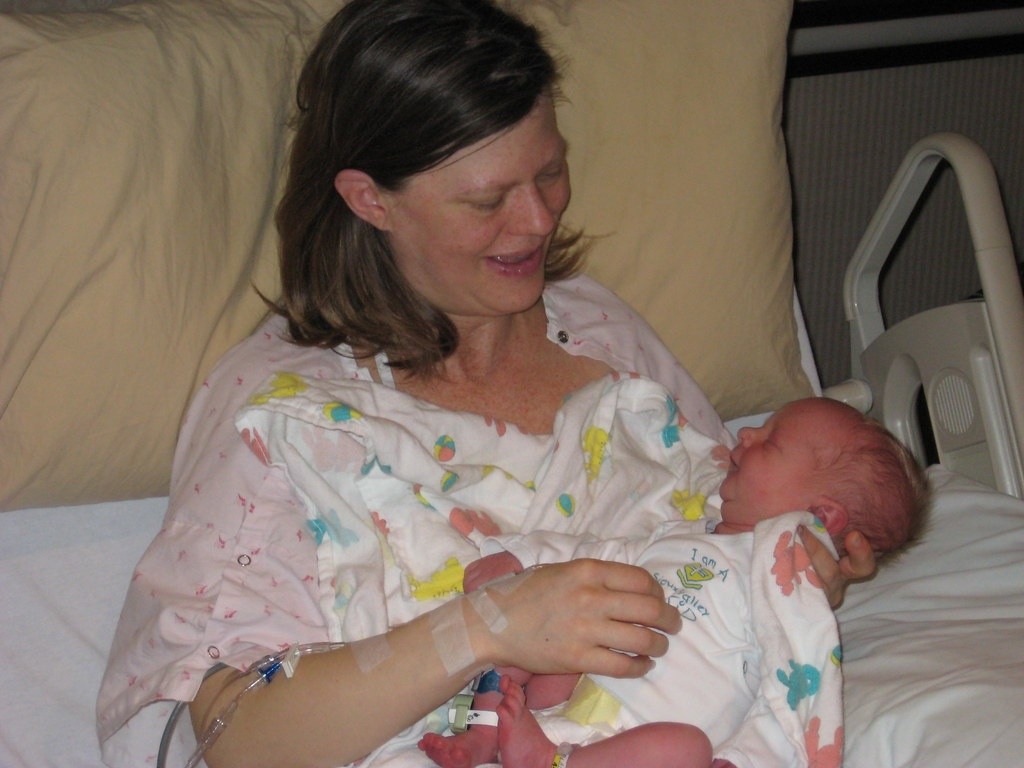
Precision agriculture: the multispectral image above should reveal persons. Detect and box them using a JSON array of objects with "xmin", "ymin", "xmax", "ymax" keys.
[
  {"xmin": 418, "ymin": 396, "xmax": 934, "ymax": 768},
  {"xmin": 98, "ymin": 5, "xmax": 878, "ymax": 768}
]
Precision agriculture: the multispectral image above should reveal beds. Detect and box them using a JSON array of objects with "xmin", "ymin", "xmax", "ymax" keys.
[{"xmin": 1, "ymin": 411, "xmax": 1024, "ymax": 767}]
